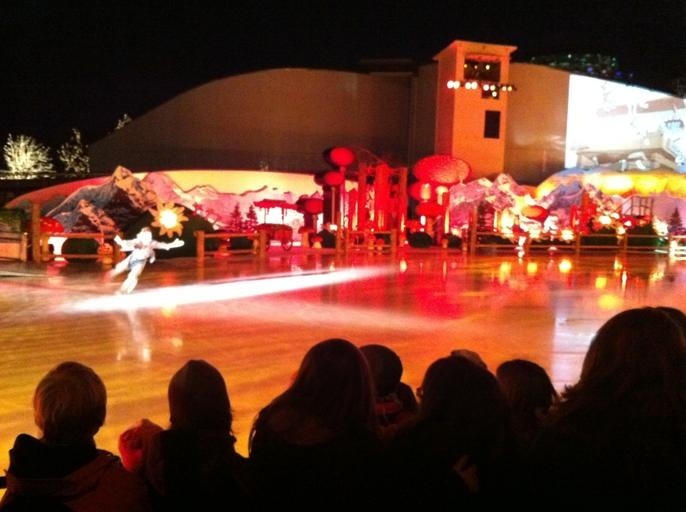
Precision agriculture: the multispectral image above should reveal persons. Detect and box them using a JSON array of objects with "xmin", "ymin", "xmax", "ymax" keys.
[
  {"xmin": 1, "ymin": 306, "xmax": 686, "ymax": 511},
  {"xmin": 100, "ymin": 227, "xmax": 186, "ymax": 296}
]
